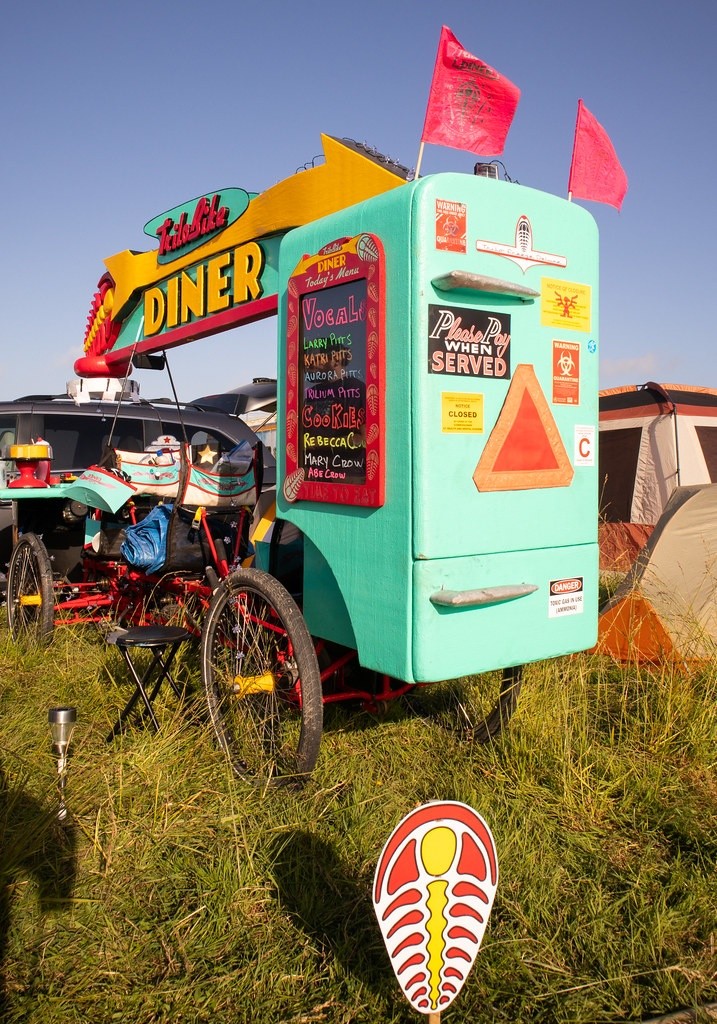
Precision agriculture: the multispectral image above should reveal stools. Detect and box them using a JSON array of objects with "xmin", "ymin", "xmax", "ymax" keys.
[{"xmin": 106, "ymin": 625, "xmax": 205, "ymax": 743}]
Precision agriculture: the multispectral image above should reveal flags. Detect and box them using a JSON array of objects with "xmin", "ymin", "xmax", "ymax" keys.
[
  {"xmin": 568, "ymin": 99, "xmax": 628, "ymax": 215},
  {"xmin": 420, "ymin": 25, "xmax": 521, "ymax": 157}
]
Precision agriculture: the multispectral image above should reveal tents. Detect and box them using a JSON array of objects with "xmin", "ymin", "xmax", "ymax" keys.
[{"xmin": 584, "ymin": 380, "xmax": 717, "ymax": 662}]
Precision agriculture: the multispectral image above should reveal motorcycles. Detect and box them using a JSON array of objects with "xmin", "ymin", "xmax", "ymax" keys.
[{"xmin": 8, "ymin": 130, "xmax": 600, "ymax": 790}]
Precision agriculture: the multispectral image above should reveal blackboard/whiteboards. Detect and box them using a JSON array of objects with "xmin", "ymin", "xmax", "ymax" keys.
[{"xmin": 283, "ymin": 231, "xmax": 386, "ymax": 509}]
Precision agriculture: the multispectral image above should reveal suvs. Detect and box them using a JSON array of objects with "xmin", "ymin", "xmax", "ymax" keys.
[{"xmin": 0, "ymin": 378, "xmax": 278, "ymax": 551}]
